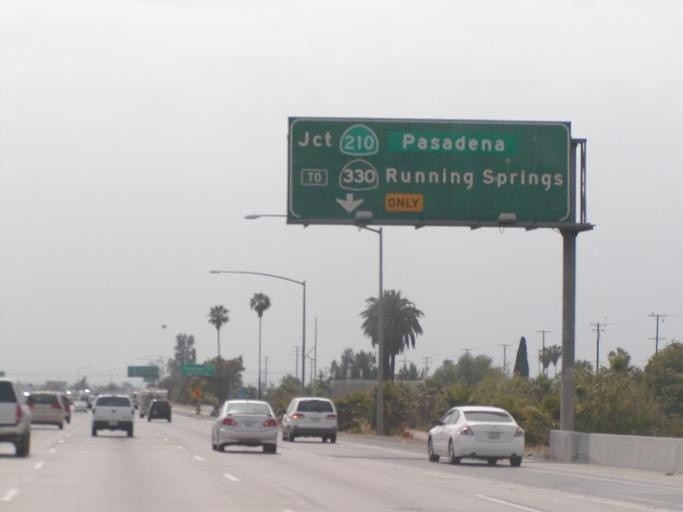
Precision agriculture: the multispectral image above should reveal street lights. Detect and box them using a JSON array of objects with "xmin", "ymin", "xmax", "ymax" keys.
[
  {"xmin": 242, "ymin": 212, "xmax": 389, "ymax": 439},
  {"xmin": 209, "ymin": 267, "xmax": 306, "ymax": 397},
  {"xmin": 135, "ymin": 354, "xmax": 165, "ymax": 390},
  {"xmin": 161, "ymin": 323, "xmax": 189, "ymax": 412}
]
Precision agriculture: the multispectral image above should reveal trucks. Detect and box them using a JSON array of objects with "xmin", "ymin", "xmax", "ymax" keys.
[{"xmin": 137, "ymin": 389, "xmax": 168, "ymax": 418}]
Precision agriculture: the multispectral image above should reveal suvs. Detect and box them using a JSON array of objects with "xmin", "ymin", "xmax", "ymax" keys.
[{"xmin": 0, "ymin": 377, "xmax": 35, "ymax": 458}]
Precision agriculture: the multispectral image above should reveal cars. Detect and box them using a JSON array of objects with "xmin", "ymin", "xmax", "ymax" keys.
[
  {"xmin": 281, "ymin": 395, "xmax": 338, "ymax": 443},
  {"xmin": 210, "ymin": 397, "xmax": 280, "ymax": 456},
  {"xmin": 147, "ymin": 398, "xmax": 174, "ymax": 424},
  {"xmin": 427, "ymin": 404, "xmax": 526, "ymax": 469},
  {"xmin": 24, "ymin": 389, "xmax": 92, "ymax": 430},
  {"xmin": 90, "ymin": 393, "xmax": 135, "ymax": 441}
]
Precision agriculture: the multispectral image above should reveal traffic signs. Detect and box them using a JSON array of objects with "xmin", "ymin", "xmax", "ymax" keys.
[
  {"xmin": 179, "ymin": 363, "xmax": 214, "ymax": 378},
  {"xmin": 287, "ymin": 115, "xmax": 573, "ymax": 229},
  {"xmin": 127, "ymin": 365, "xmax": 158, "ymax": 378}
]
